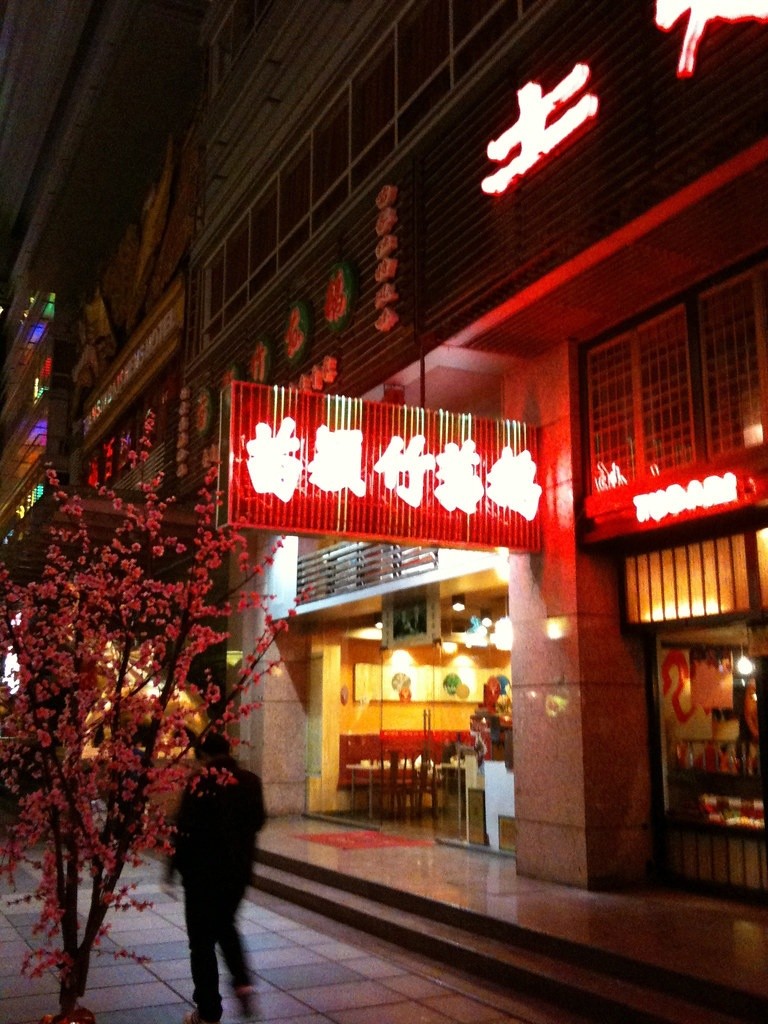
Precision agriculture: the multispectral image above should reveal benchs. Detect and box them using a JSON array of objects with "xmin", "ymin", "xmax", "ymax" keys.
[{"xmin": 339, "ymin": 729, "xmax": 492, "ymax": 790}]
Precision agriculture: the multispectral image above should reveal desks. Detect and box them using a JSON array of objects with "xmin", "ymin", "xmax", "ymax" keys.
[
  {"xmin": 345, "ymin": 763, "xmax": 443, "ymax": 820},
  {"xmin": 442, "ymin": 763, "xmax": 466, "ymax": 796}
]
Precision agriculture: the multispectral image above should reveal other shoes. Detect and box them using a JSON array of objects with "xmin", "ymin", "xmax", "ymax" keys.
[
  {"xmin": 232, "ymin": 975, "xmax": 252, "ymax": 995},
  {"xmin": 183, "ymin": 1010, "xmax": 219, "ymax": 1024}
]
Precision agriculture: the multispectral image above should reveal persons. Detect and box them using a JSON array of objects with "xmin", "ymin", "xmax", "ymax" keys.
[{"xmin": 163, "ymin": 730, "xmax": 268, "ymax": 1024}]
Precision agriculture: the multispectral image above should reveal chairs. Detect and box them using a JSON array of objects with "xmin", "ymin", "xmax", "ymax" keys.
[{"xmin": 366, "ymin": 749, "xmax": 439, "ymax": 822}]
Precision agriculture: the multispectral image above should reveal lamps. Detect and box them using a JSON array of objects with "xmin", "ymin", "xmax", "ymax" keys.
[
  {"xmin": 481, "ymin": 607, "xmax": 492, "ymax": 628},
  {"xmin": 451, "ymin": 593, "xmax": 465, "ymax": 612}
]
